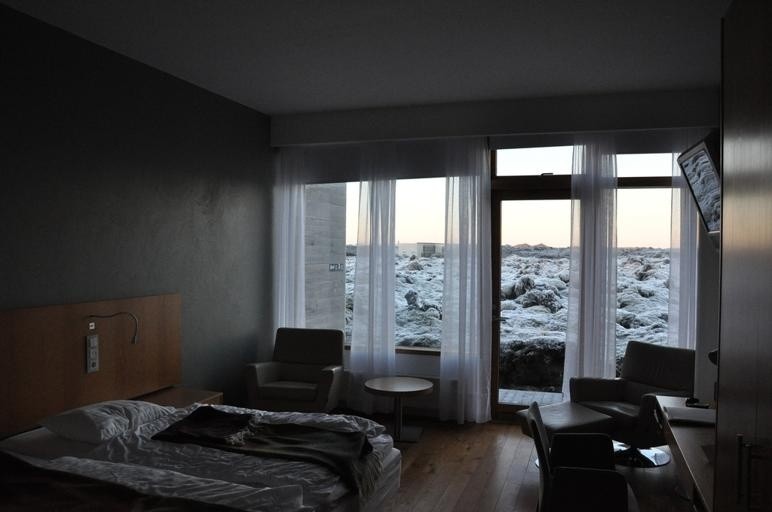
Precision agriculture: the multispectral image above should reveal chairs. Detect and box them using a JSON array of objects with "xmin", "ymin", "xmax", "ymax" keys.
[
  {"xmin": 567, "ymin": 340, "xmax": 699, "ymax": 467},
  {"xmin": 524, "ymin": 401, "xmax": 630, "ymax": 512},
  {"xmin": 243, "ymin": 326, "xmax": 344, "ymax": 413}
]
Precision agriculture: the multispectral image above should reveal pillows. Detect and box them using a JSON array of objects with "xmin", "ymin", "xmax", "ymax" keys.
[{"xmin": 39, "ymin": 399, "xmax": 174, "ymax": 443}]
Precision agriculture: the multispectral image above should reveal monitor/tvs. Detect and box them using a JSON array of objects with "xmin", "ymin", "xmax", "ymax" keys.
[{"xmin": 677, "ymin": 128, "xmax": 722, "ymax": 234}]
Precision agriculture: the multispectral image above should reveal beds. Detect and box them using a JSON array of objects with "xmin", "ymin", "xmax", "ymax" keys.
[{"xmin": 0, "ymin": 398, "xmax": 405, "ymax": 512}]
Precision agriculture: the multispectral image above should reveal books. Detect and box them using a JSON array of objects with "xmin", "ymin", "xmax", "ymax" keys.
[{"xmin": 663, "ymin": 405, "xmax": 716, "ymax": 426}]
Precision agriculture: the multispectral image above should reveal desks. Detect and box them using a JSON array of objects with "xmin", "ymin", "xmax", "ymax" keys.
[{"xmin": 644, "ymin": 390, "xmax": 717, "ymax": 511}]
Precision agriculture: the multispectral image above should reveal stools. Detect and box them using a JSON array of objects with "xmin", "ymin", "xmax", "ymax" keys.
[
  {"xmin": 516, "ymin": 402, "xmax": 613, "ymax": 469},
  {"xmin": 362, "ymin": 376, "xmax": 435, "ymax": 445}
]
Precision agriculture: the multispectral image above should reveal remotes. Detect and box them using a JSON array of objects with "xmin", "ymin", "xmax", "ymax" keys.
[{"xmin": 687, "ymin": 402, "xmax": 710, "ymax": 409}]
